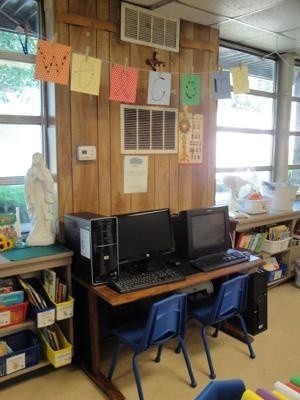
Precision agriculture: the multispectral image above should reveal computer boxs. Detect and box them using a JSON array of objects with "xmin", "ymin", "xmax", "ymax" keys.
[
  {"xmin": 63, "ymin": 211, "xmax": 120, "ymax": 286},
  {"xmin": 213, "ymin": 272, "xmax": 268, "ymax": 336}
]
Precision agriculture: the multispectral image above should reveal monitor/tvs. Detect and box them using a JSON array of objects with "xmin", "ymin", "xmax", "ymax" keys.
[
  {"xmin": 172, "ymin": 204, "xmax": 229, "ymax": 259},
  {"xmin": 110, "ymin": 208, "xmax": 176, "ymax": 273}
]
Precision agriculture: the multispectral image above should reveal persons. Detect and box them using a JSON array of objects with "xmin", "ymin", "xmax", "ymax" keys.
[{"xmin": 24, "ymin": 151, "xmax": 56, "ymax": 247}]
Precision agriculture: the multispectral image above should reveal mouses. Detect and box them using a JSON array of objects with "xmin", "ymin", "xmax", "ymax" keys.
[{"xmin": 243, "ymin": 251, "xmax": 250, "ymax": 256}]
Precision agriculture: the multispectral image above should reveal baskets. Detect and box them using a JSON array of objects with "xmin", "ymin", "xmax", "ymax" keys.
[
  {"xmin": 267, "ymin": 261, "xmax": 288, "ymax": 282},
  {"xmin": 0, "ymin": 299, "xmax": 29, "ymax": 329},
  {"xmin": 0, "ymin": 329, "xmax": 42, "ymax": 378},
  {"xmin": 261, "ymin": 237, "xmax": 292, "ymax": 254}
]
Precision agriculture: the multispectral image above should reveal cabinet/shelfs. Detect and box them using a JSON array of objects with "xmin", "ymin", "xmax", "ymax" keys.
[
  {"xmin": 0, "ymin": 243, "xmax": 74, "ymax": 390},
  {"xmin": 228, "ymin": 201, "xmax": 300, "ymax": 287}
]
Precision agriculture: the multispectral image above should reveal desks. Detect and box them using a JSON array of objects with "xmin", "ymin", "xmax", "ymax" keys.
[{"xmin": 72, "ymin": 252, "xmax": 266, "ymax": 400}]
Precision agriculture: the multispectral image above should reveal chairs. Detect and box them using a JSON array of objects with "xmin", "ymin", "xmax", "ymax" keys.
[
  {"xmin": 108, "ymin": 294, "xmax": 197, "ymax": 400},
  {"xmin": 187, "ymin": 275, "xmax": 255, "ymax": 379}
]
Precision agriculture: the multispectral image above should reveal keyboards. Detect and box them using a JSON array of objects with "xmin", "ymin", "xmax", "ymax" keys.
[
  {"xmin": 110, "ymin": 266, "xmax": 185, "ymax": 294},
  {"xmin": 190, "ymin": 250, "xmax": 248, "ymax": 272}
]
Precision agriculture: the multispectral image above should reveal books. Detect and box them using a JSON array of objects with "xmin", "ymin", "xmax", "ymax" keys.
[
  {"xmin": 44, "ymin": 269, "xmax": 67, "ymax": 303},
  {"xmin": 234, "ymin": 224, "xmax": 290, "ymax": 254},
  {"xmin": 19, "ymin": 278, "xmax": 47, "ymax": 310},
  {"xmin": 42, "ymin": 327, "xmax": 59, "ymax": 350}
]
